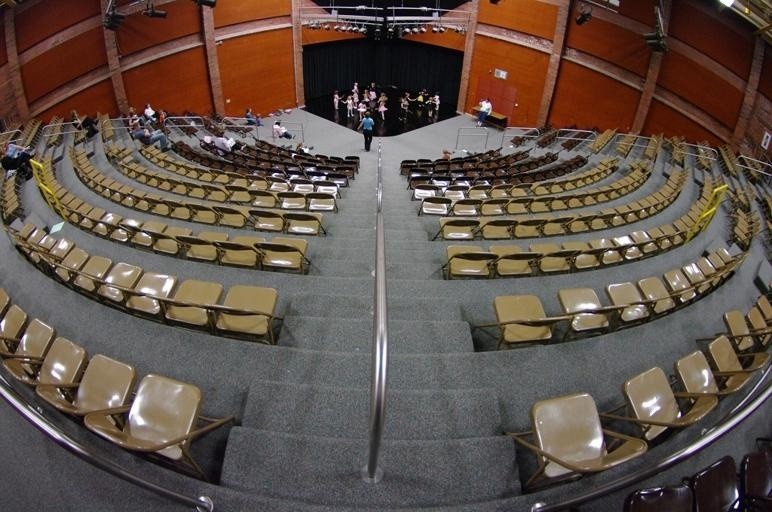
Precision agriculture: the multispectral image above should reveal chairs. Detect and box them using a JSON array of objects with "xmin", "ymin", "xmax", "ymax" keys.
[{"xmin": 623, "ymin": 437, "xmax": 772, "ymax": 512}]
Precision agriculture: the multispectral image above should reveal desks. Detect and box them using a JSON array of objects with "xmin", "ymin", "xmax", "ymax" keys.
[{"xmin": 471, "ymin": 105, "xmax": 508, "ymax": 132}]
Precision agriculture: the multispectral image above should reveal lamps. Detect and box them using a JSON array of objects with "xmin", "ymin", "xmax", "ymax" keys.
[
  {"xmin": 193, "ymin": 0, "xmax": 217, "ymax": 8},
  {"xmin": 643, "ymin": 29, "xmax": 666, "ymax": 49},
  {"xmin": 102, "ymin": 0, "xmax": 126, "ymax": 31},
  {"xmin": 301, "ymin": 21, "xmax": 467, "ymax": 36},
  {"xmin": 144, "ymin": 0, "xmax": 169, "ymax": 18},
  {"xmin": 576, "ymin": 4, "xmax": 592, "ymax": 26}
]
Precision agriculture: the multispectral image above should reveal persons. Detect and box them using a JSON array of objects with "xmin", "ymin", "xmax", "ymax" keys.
[
  {"xmin": 272, "ymin": 120, "xmax": 296, "ymax": 140},
  {"xmin": 332, "ymin": 80, "xmax": 441, "ymax": 121},
  {"xmin": 0, "ymin": 103, "xmax": 171, "ymax": 174},
  {"xmin": 215, "ymin": 129, "xmax": 247, "ymax": 157},
  {"xmin": 477, "ymin": 98, "xmax": 493, "ymax": 127},
  {"xmin": 245, "ymin": 107, "xmax": 264, "ymax": 126},
  {"xmin": 357, "ymin": 111, "xmax": 375, "ymax": 152}
]
